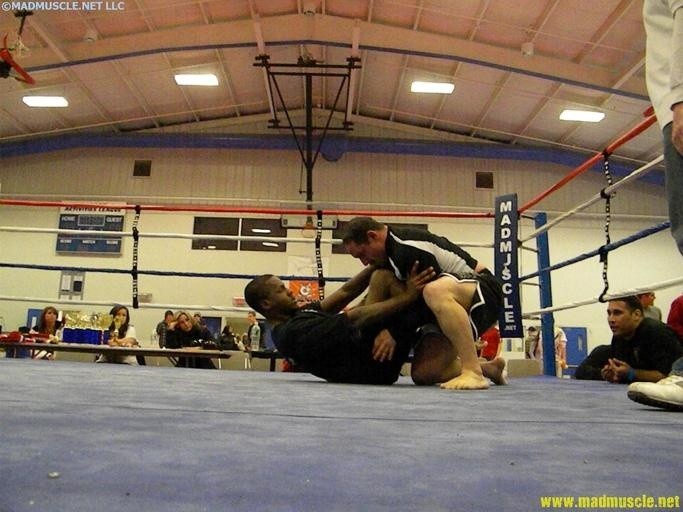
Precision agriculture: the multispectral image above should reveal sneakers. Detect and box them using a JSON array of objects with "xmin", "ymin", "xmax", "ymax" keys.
[{"xmin": 627, "ymin": 375, "xmax": 683, "ymax": 408}]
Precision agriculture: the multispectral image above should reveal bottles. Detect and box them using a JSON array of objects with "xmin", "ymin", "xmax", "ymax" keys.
[
  {"xmin": 249, "ymin": 321, "xmax": 259, "ymax": 351},
  {"xmin": 150, "ymin": 327, "xmax": 158, "ymax": 348}
]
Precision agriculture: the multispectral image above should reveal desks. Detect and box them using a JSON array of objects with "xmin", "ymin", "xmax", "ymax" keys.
[
  {"xmin": 241, "ymin": 346, "xmax": 286, "ymax": 374},
  {"xmin": 0, "ymin": 333, "xmax": 233, "ymax": 371}
]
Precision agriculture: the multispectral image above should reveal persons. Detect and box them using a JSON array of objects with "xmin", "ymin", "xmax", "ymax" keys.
[
  {"xmin": 343, "ymin": 216, "xmax": 507, "ymax": 390},
  {"xmin": 95, "ymin": 306, "xmax": 141, "ymax": 366},
  {"xmin": 244, "ymin": 259, "xmax": 437, "ymax": 385},
  {"xmin": 524, "ymin": 290, "xmax": 683, "ymax": 383},
  {"xmin": 152, "ymin": 311, "xmax": 262, "ymax": 370},
  {"xmin": 480, "ymin": 321, "xmax": 502, "ymax": 361},
  {"xmin": 0, "ymin": 307, "xmax": 69, "ymax": 360},
  {"xmin": 627, "ymin": 0, "xmax": 683, "ymax": 410}
]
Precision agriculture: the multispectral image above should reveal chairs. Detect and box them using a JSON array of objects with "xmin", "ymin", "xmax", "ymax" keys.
[{"xmin": 506, "ymin": 359, "xmax": 540, "ymax": 378}]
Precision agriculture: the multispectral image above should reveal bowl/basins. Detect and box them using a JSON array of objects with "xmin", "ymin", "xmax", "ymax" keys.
[{"xmin": 29, "ymin": 334, "xmax": 52, "ymax": 343}]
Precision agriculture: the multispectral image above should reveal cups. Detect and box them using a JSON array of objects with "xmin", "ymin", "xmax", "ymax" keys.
[{"xmin": 135, "ymin": 336, "xmax": 143, "ymax": 348}]
